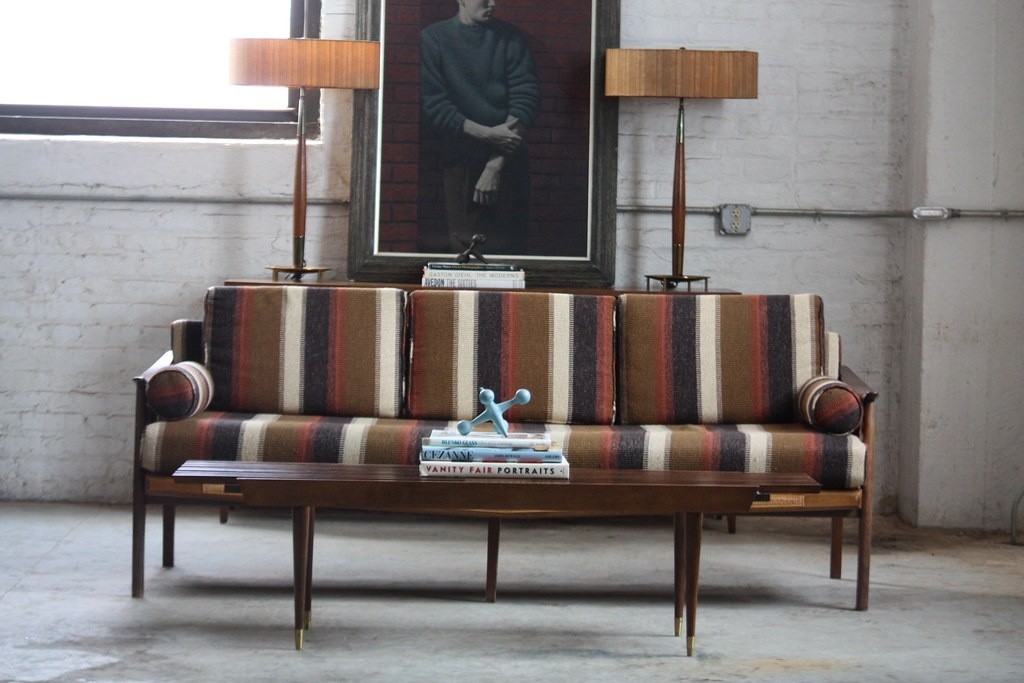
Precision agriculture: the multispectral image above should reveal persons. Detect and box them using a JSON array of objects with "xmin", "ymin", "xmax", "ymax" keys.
[{"xmin": 421, "ymin": 0, "xmax": 541, "ymax": 255}]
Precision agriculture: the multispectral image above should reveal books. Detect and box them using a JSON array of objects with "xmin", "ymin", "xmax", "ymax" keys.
[
  {"xmin": 421, "ymin": 262, "xmax": 525, "ymax": 288},
  {"xmin": 419, "ymin": 430, "xmax": 570, "ymax": 478}
]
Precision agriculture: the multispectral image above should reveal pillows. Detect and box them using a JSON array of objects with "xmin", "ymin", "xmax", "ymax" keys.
[
  {"xmin": 407, "ymin": 289, "xmax": 617, "ymax": 425},
  {"xmin": 202, "ymin": 284, "xmax": 406, "ymax": 418},
  {"xmin": 617, "ymin": 291, "xmax": 827, "ymax": 426}
]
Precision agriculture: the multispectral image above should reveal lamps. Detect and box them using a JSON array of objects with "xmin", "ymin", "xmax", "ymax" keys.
[
  {"xmin": 233, "ymin": 38, "xmax": 379, "ymax": 280},
  {"xmin": 605, "ymin": 47, "xmax": 759, "ymax": 290}
]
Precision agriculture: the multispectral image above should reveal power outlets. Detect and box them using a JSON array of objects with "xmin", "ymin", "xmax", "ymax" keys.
[{"xmin": 718, "ymin": 202, "xmax": 752, "ymax": 235}]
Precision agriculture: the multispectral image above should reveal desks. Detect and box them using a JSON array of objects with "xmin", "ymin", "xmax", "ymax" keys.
[
  {"xmin": 173, "ymin": 460, "xmax": 823, "ymax": 657},
  {"xmin": 219, "ymin": 278, "xmax": 743, "ymax": 535}
]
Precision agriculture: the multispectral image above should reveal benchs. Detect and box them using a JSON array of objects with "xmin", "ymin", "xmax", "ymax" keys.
[{"xmin": 131, "ymin": 317, "xmax": 881, "ymax": 612}]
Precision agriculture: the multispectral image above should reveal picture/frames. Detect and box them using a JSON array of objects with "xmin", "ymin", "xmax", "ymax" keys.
[{"xmin": 348, "ymin": 0, "xmax": 619, "ymax": 286}]
